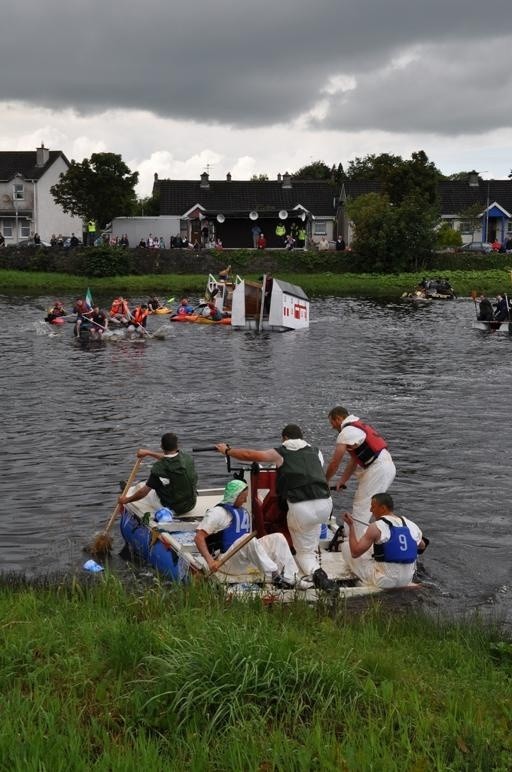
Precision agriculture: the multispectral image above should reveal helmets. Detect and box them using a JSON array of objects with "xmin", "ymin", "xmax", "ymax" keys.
[
  {"xmin": 182, "ymin": 299, "xmax": 188, "ymax": 305},
  {"xmin": 210, "ymin": 310, "xmax": 217, "ymax": 316}
]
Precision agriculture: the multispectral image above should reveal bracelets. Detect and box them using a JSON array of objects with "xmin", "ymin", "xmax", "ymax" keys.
[{"xmin": 225, "ymin": 447, "xmax": 230, "ymax": 455}]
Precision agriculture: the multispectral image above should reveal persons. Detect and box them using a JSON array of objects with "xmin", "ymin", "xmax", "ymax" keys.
[
  {"xmin": 341, "ymin": 493, "xmax": 425, "ymax": 587},
  {"xmin": 324, "ymin": 406, "xmax": 397, "ymax": 555},
  {"xmin": 28, "ymin": 231, "xmax": 223, "ymax": 251},
  {"xmin": 421, "ymin": 276, "xmax": 455, "ymax": 300},
  {"xmin": 118, "ymin": 433, "xmax": 198, "ymax": 514},
  {"xmin": 478, "ymin": 295, "xmax": 511, "ymax": 321},
  {"xmin": 259, "ymin": 221, "xmax": 345, "ymax": 250},
  {"xmin": 0, "ymin": 233, "xmax": 5, "ymax": 247},
  {"xmin": 261, "ymin": 273, "xmax": 273, "ymax": 313},
  {"xmin": 489, "ymin": 237, "xmax": 512, "ymax": 253},
  {"xmin": 194, "ymin": 479, "xmax": 315, "ymax": 589},
  {"xmin": 216, "ymin": 424, "xmax": 333, "ymax": 590},
  {"xmin": 44, "ymin": 266, "xmax": 231, "ymax": 341}
]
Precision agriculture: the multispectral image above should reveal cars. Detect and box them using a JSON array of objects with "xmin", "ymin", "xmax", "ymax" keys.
[
  {"xmin": 457, "ymin": 241, "xmax": 499, "ymax": 254},
  {"xmin": 7, "ymin": 238, "xmax": 52, "ymax": 249}
]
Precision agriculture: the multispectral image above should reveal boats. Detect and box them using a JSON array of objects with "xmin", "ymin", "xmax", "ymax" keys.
[
  {"xmin": 400, "ymin": 287, "xmax": 456, "ymax": 304},
  {"xmin": 118, "ymin": 477, "xmax": 421, "ymax": 603},
  {"xmin": 43, "ymin": 301, "xmax": 231, "ymax": 340},
  {"xmin": 471, "ymin": 315, "xmax": 512, "ymax": 332}
]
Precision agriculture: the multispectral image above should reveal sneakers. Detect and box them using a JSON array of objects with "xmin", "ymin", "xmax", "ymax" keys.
[
  {"xmin": 313, "ymin": 568, "xmax": 332, "ymax": 593},
  {"xmin": 293, "ymin": 579, "xmax": 314, "ymax": 590},
  {"xmin": 274, "ymin": 574, "xmax": 294, "ymax": 589}
]
